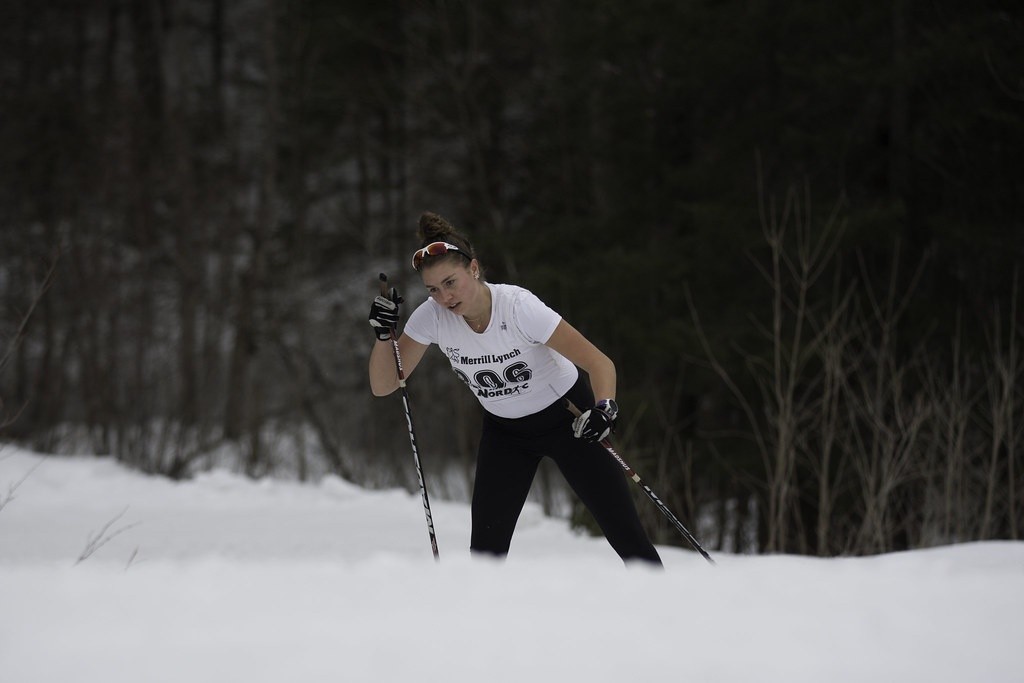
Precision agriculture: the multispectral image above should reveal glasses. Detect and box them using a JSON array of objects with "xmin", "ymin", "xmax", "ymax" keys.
[{"xmin": 412, "ymin": 242, "xmax": 473, "ymax": 272}]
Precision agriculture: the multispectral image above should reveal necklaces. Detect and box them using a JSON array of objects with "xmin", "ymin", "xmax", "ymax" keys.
[{"xmin": 466, "ymin": 310, "xmax": 489, "ymax": 334}]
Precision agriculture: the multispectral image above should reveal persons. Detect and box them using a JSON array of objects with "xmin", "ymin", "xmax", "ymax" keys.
[{"xmin": 368, "ymin": 213, "xmax": 664, "ymax": 570}]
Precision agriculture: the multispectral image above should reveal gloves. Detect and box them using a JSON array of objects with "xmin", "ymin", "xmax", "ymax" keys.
[
  {"xmin": 572, "ymin": 399, "xmax": 618, "ymax": 448},
  {"xmin": 369, "ymin": 287, "xmax": 404, "ymax": 341}
]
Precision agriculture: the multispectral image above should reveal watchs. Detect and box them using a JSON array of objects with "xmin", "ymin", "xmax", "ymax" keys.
[{"xmin": 597, "ymin": 399, "xmax": 618, "ymax": 414}]
